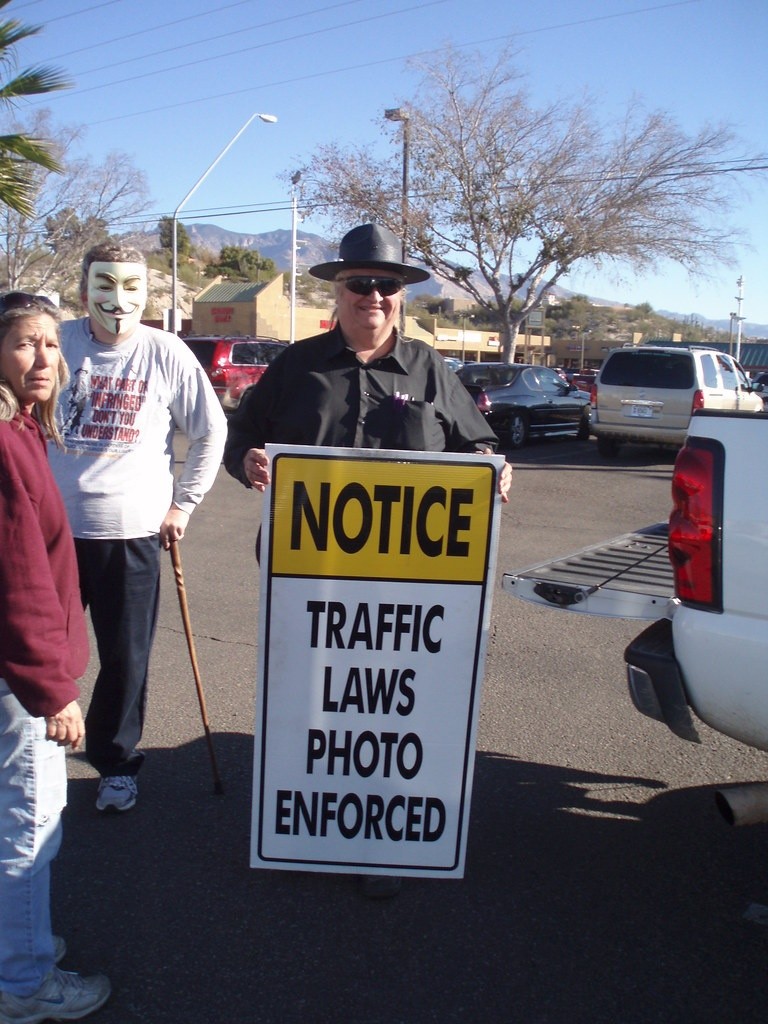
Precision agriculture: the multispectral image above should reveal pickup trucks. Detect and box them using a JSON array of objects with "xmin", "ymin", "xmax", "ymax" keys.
[{"xmin": 623, "ymin": 410, "xmax": 768, "ymax": 750}]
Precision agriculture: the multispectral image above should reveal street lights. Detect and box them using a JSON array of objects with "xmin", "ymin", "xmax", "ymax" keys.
[
  {"xmin": 454, "ymin": 313, "xmax": 476, "ymax": 363},
  {"xmin": 571, "ymin": 327, "xmax": 592, "ymax": 368},
  {"xmin": 172, "ymin": 113, "xmax": 277, "ymax": 334},
  {"xmin": 385, "ymin": 105, "xmax": 409, "ymax": 332}
]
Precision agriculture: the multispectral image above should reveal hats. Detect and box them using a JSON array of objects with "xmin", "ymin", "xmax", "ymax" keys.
[{"xmin": 308, "ymin": 224, "xmax": 431, "ymax": 284}]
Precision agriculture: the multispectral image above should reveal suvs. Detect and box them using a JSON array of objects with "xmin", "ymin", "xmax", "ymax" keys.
[
  {"xmin": 591, "ymin": 342, "xmax": 768, "ymax": 459},
  {"xmin": 174, "ymin": 335, "xmax": 291, "ymax": 464}
]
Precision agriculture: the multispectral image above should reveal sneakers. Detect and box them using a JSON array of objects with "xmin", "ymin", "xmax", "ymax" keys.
[
  {"xmin": 49, "ymin": 933, "xmax": 67, "ymax": 965},
  {"xmin": 95, "ymin": 776, "xmax": 138, "ymax": 813},
  {"xmin": 0, "ymin": 969, "xmax": 112, "ymax": 1024}
]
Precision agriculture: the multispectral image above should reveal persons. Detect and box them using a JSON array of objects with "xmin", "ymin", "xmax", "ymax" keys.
[
  {"xmin": 0, "ymin": 291, "xmax": 113, "ymax": 1024},
  {"xmin": 225, "ymin": 223, "xmax": 513, "ymax": 896},
  {"xmin": 40, "ymin": 241, "xmax": 228, "ymax": 812}
]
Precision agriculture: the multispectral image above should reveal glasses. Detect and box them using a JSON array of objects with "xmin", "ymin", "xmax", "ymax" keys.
[
  {"xmin": 336, "ymin": 275, "xmax": 405, "ymax": 297},
  {"xmin": 0, "ymin": 292, "xmax": 56, "ymax": 318}
]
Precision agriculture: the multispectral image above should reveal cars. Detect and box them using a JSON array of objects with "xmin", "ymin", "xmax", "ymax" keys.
[{"xmin": 440, "ymin": 357, "xmax": 599, "ymax": 448}]
